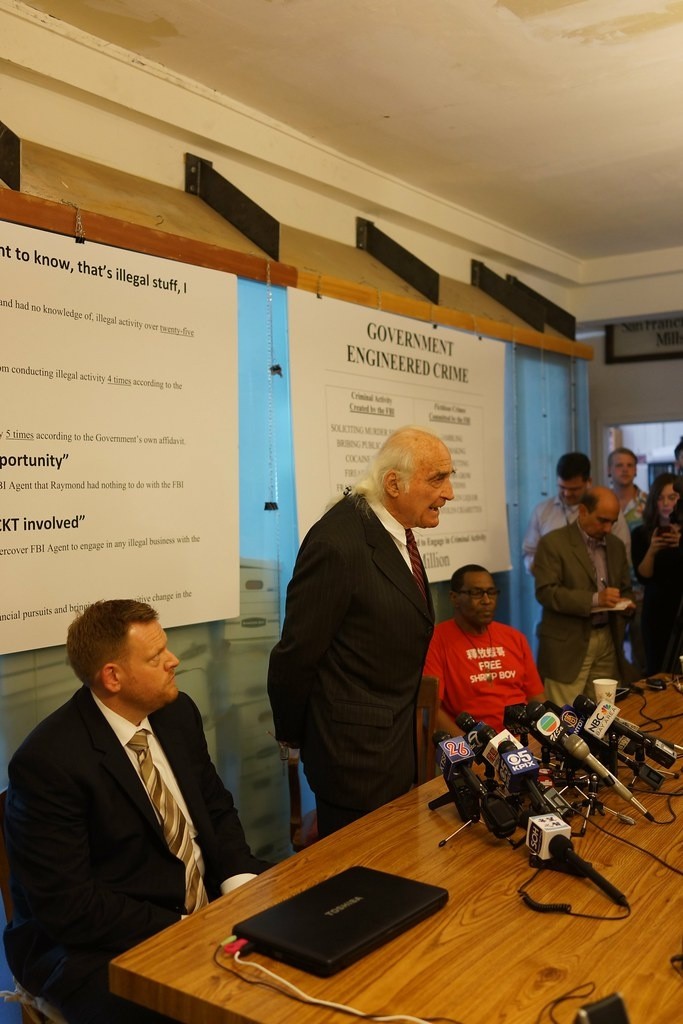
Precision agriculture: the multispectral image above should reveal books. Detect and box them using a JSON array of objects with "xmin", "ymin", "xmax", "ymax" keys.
[{"xmin": 592, "ymin": 599, "xmax": 636, "ymax": 612}]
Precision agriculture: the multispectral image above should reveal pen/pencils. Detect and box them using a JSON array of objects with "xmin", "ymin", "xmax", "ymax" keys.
[{"xmin": 600, "ymin": 577, "xmax": 607, "ymax": 588}]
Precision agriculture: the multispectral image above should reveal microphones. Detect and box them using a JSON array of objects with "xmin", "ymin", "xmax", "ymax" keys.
[{"xmin": 432, "ymin": 693, "xmax": 683, "ymax": 906}]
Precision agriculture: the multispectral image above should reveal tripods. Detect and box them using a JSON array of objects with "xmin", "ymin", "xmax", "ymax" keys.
[
  {"xmin": 571, "ymin": 772, "xmax": 636, "ymax": 836},
  {"xmin": 558, "ymin": 752, "xmax": 588, "ymax": 800},
  {"xmin": 439, "ymin": 789, "xmax": 517, "ymax": 847},
  {"xmin": 617, "ymin": 740, "xmax": 679, "ymax": 789},
  {"xmin": 482, "ymin": 761, "xmax": 507, "ymax": 797}
]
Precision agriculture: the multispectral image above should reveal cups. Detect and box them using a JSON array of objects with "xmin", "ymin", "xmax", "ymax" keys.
[{"xmin": 593, "ymin": 678, "xmax": 618, "ymax": 707}]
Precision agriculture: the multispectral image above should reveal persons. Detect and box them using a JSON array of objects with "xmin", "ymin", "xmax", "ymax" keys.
[
  {"xmin": 531, "ymin": 488, "xmax": 635, "ymax": 708},
  {"xmin": 522, "ymin": 451, "xmax": 632, "ymax": 574},
  {"xmin": 0, "ymin": 598, "xmax": 261, "ymax": 1024},
  {"xmin": 266, "ymin": 430, "xmax": 457, "ymax": 836},
  {"xmin": 424, "ymin": 565, "xmax": 546, "ymax": 738},
  {"xmin": 607, "ymin": 438, "xmax": 683, "ymax": 675}
]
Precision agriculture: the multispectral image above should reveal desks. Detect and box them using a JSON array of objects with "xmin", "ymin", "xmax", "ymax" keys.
[{"xmin": 109, "ymin": 671, "xmax": 683, "ymax": 1024}]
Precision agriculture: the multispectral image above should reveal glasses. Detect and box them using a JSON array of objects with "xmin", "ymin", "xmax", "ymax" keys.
[{"xmin": 456, "ymin": 588, "xmax": 500, "ymax": 599}]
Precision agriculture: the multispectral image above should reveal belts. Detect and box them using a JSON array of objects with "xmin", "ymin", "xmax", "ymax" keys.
[{"xmin": 592, "ymin": 622, "xmax": 608, "ymax": 630}]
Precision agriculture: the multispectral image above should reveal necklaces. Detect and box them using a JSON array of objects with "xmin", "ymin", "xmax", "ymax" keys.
[{"xmin": 455, "ymin": 619, "xmax": 492, "ymax": 681}]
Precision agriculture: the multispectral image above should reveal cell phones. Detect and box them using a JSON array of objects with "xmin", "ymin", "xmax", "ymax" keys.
[{"xmin": 657, "ymin": 525, "xmax": 672, "ymax": 536}]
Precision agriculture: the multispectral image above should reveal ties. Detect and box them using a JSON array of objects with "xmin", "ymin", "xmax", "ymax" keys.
[
  {"xmin": 406, "ymin": 528, "xmax": 427, "ymax": 601},
  {"xmin": 126, "ymin": 729, "xmax": 209, "ymax": 916}
]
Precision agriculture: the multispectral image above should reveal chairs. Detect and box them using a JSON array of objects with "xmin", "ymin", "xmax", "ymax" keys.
[
  {"xmin": 287, "ymin": 678, "xmax": 441, "ymax": 853},
  {"xmin": 0, "ymin": 786, "xmax": 69, "ymax": 1024}
]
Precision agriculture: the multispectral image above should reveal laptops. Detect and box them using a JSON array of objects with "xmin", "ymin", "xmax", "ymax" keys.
[{"xmin": 232, "ymin": 864, "xmax": 450, "ymax": 979}]
